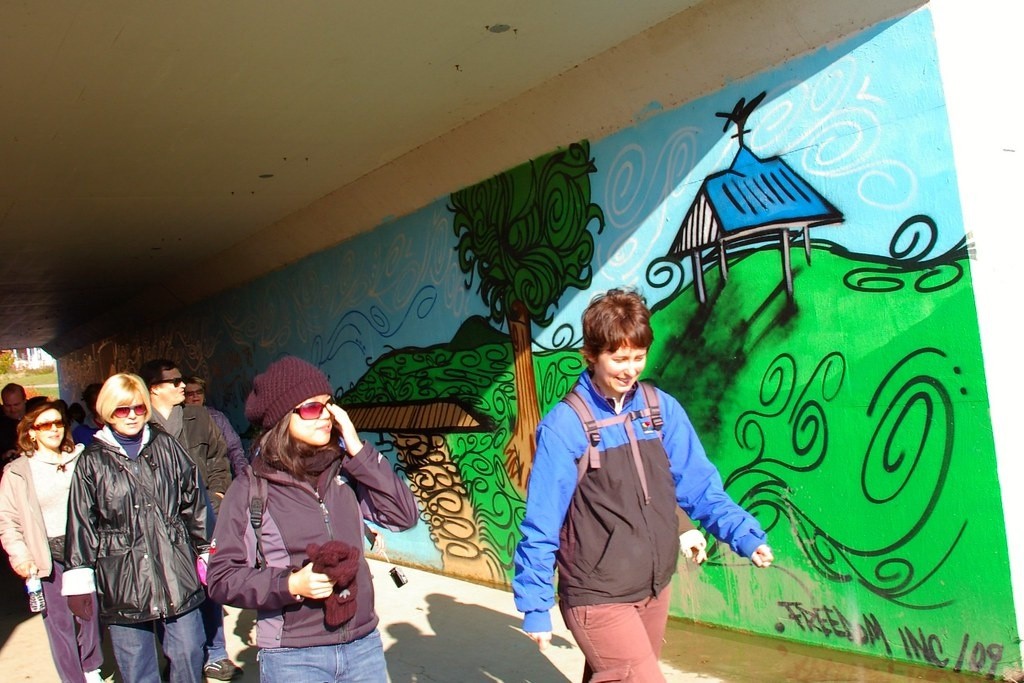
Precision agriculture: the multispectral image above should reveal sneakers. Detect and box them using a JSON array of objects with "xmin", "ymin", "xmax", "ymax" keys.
[{"xmin": 204, "ymin": 657, "xmax": 245, "ymax": 679}]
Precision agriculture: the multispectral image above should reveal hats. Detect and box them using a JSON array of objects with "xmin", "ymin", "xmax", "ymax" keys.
[{"xmin": 245, "ymin": 355, "xmax": 334, "ymax": 429}]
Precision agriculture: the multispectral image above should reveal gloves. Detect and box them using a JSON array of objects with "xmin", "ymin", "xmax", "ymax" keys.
[
  {"xmin": 67, "ymin": 593, "xmax": 93, "ymax": 621},
  {"xmin": 291, "ymin": 540, "xmax": 359, "ymax": 630}
]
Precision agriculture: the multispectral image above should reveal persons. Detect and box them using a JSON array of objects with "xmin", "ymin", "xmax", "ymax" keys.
[
  {"xmin": 209, "ymin": 355, "xmax": 419, "ymax": 683},
  {"xmin": 60, "ymin": 373, "xmax": 213, "ymax": 683},
  {"xmin": 0, "ymin": 401, "xmax": 107, "ymax": 683},
  {"xmin": 0, "ymin": 359, "xmax": 264, "ymax": 683},
  {"xmin": 581, "ymin": 502, "xmax": 709, "ymax": 683},
  {"xmin": 511, "ymin": 288, "xmax": 775, "ymax": 683}
]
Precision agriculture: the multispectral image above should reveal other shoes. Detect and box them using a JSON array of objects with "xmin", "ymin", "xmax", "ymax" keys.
[{"xmin": 84, "ymin": 667, "xmax": 107, "ymax": 683}]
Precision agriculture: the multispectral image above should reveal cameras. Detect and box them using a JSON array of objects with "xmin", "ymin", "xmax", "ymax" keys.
[{"xmin": 390, "ymin": 566, "xmax": 408, "ymax": 588}]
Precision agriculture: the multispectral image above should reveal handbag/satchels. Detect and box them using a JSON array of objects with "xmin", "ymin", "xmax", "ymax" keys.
[{"xmin": 197, "ymin": 553, "xmax": 208, "ymax": 585}]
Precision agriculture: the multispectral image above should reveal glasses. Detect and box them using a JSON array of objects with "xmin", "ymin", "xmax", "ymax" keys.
[
  {"xmin": 292, "ymin": 397, "xmax": 334, "ymax": 421},
  {"xmin": 186, "ymin": 388, "xmax": 206, "ymax": 396},
  {"xmin": 113, "ymin": 403, "xmax": 146, "ymax": 417},
  {"xmin": 150, "ymin": 375, "xmax": 187, "ymax": 388},
  {"xmin": 30, "ymin": 419, "xmax": 66, "ymax": 431}
]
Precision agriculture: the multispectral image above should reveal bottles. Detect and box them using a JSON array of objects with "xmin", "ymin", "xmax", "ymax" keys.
[{"xmin": 25, "ymin": 568, "xmax": 46, "ymax": 612}]
